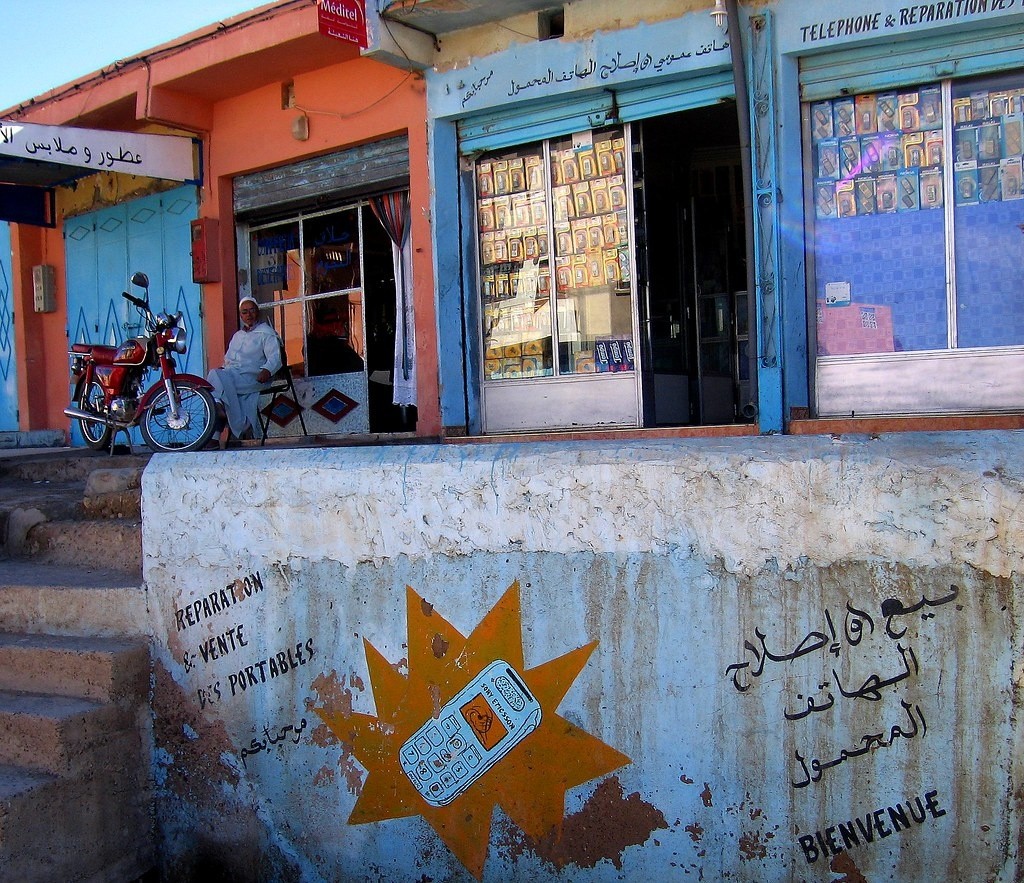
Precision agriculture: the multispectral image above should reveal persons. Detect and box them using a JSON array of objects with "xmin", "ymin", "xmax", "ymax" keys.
[{"xmin": 205, "ymin": 296, "xmax": 285, "ymax": 451}]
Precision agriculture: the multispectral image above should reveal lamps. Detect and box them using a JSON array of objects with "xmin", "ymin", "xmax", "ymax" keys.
[
  {"xmin": 292, "ymin": 114, "xmax": 309, "ymax": 141},
  {"xmin": 712, "ymin": 0, "xmax": 727, "ymax": 27}
]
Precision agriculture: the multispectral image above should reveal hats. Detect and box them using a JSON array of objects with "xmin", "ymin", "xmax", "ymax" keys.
[{"xmin": 239, "ymin": 296, "xmax": 259, "ymax": 311}]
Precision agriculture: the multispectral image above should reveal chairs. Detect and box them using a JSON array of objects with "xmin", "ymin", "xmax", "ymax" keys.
[{"xmin": 223, "ymin": 341, "xmax": 307, "ymax": 448}]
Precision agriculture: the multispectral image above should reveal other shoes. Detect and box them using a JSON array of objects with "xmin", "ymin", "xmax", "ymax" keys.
[
  {"xmin": 216, "ymin": 401, "xmax": 226, "ymax": 423},
  {"xmin": 202, "ymin": 439, "xmax": 220, "ymax": 450}
]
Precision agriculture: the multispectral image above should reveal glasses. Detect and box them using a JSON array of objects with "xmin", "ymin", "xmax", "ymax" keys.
[{"xmin": 239, "ymin": 308, "xmax": 257, "ymax": 314}]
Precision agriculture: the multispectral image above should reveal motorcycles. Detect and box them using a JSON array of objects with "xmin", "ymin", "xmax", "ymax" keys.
[{"xmin": 64, "ymin": 270, "xmax": 219, "ymax": 455}]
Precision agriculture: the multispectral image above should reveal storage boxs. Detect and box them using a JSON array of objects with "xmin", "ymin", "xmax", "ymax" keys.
[
  {"xmin": 476, "ymin": 137, "xmax": 633, "ymax": 374},
  {"xmin": 810, "ymin": 87, "xmax": 1024, "ymax": 220}
]
